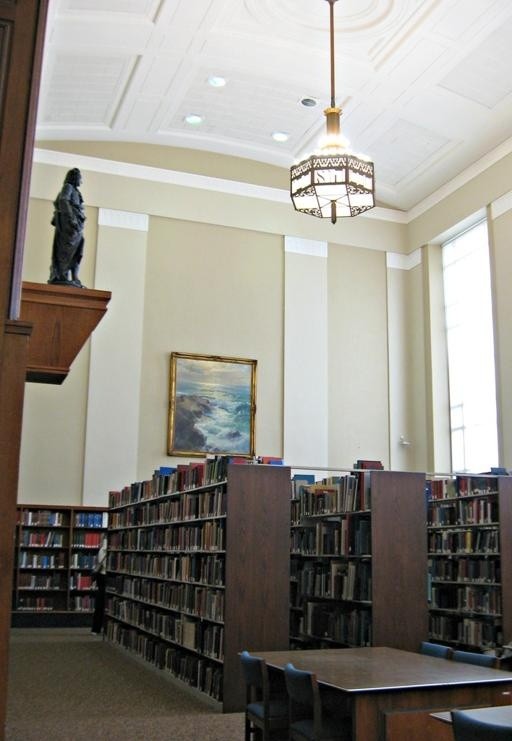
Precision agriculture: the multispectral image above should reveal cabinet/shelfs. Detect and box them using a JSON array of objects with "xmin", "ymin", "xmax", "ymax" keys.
[
  {"xmin": 424, "ymin": 468, "xmax": 512, "ymax": 664},
  {"xmin": 11, "ymin": 502, "xmax": 110, "ymax": 628},
  {"xmin": 288, "ymin": 460, "xmax": 429, "ymax": 650},
  {"xmin": 105, "ymin": 457, "xmax": 293, "ymax": 713}
]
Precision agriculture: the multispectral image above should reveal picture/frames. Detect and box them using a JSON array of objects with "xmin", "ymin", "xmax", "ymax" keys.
[{"xmin": 166, "ymin": 351, "xmax": 260, "ymax": 460}]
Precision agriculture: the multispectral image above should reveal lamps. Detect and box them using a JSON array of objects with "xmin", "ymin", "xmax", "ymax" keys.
[{"xmin": 289, "ymin": 0, "xmax": 378, "ymax": 226}]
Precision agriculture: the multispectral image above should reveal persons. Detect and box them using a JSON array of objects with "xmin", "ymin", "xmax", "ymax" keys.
[{"xmin": 52, "ymin": 168, "xmax": 86, "ymax": 283}]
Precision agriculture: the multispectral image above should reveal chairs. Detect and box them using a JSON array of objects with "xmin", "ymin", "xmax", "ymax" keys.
[
  {"xmin": 284, "ymin": 660, "xmax": 352, "ymax": 739},
  {"xmin": 238, "ymin": 650, "xmax": 290, "ymax": 740},
  {"xmin": 450, "ymin": 708, "xmax": 511, "ymax": 741},
  {"xmin": 452, "ymin": 649, "xmax": 499, "ymax": 670},
  {"xmin": 418, "ymin": 640, "xmax": 453, "ymax": 661}
]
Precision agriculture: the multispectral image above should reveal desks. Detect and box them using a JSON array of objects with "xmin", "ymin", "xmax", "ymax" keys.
[
  {"xmin": 428, "ymin": 704, "xmax": 512, "ymax": 725},
  {"xmin": 237, "ymin": 645, "xmax": 511, "ymax": 741}
]
